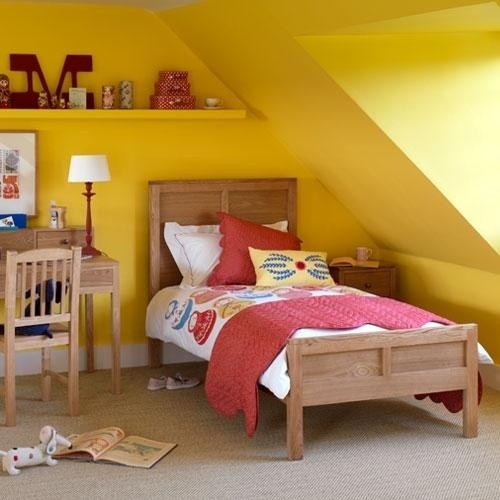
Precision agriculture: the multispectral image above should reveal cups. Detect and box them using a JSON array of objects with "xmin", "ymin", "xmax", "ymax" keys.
[
  {"xmin": 205, "ymin": 97, "xmax": 225, "ymax": 106},
  {"xmin": 355, "ymin": 247, "xmax": 373, "ymax": 261}
]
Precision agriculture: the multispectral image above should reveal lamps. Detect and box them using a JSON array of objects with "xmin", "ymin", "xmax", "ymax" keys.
[{"xmin": 68, "ymin": 155, "xmax": 111, "ymax": 256}]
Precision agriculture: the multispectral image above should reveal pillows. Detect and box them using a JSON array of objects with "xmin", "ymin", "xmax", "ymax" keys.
[
  {"xmin": 248, "ymin": 246, "xmax": 336, "ymax": 286},
  {"xmin": 164, "ymin": 220, "xmax": 288, "ymax": 289},
  {"xmin": 207, "ymin": 212, "xmax": 303, "ymax": 286}
]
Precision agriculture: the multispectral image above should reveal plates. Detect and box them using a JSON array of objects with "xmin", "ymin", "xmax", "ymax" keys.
[{"xmin": 202, "ymin": 104, "xmax": 222, "ymax": 109}]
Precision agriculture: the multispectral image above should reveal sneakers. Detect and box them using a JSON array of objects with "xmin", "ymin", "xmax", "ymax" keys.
[
  {"xmin": 167, "ymin": 374, "xmax": 200, "ymax": 389},
  {"xmin": 146, "ymin": 375, "xmax": 167, "ymax": 390}
]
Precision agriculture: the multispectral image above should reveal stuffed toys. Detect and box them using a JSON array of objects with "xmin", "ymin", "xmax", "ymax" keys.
[
  {"xmin": 0, "ymin": 278, "xmax": 68, "ymax": 339},
  {"xmin": 0, "ymin": 425, "xmax": 73, "ymax": 476}
]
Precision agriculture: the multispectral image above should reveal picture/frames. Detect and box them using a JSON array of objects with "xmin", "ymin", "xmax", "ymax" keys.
[{"xmin": 0, "ymin": 129, "xmax": 39, "ymax": 219}]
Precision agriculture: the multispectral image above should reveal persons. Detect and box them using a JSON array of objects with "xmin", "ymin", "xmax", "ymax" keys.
[{"xmin": 38, "ymin": 90, "xmax": 48, "ymax": 107}]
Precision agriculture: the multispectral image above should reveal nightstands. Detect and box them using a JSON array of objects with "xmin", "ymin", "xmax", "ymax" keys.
[{"xmin": 328, "ymin": 259, "xmax": 397, "ymax": 299}]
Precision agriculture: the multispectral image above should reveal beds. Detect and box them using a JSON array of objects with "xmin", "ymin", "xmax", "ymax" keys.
[{"xmin": 145, "ymin": 177, "xmax": 478, "ymax": 460}]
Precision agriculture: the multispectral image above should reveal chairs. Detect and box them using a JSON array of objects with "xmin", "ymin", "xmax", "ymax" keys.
[{"xmin": 0, "ymin": 245, "xmax": 82, "ymax": 426}]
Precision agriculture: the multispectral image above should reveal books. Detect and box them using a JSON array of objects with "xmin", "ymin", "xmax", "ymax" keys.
[
  {"xmin": 52, "ymin": 426, "xmax": 177, "ymax": 469},
  {"xmin": 329, "ymin": 256, "xmax": 380, "ymax": 269},
  {"xmin": 38, "ymin": 254, "xmax": 93, "ymax": 265}
]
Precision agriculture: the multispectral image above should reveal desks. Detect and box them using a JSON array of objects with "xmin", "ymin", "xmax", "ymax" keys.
[{"xmin": 0, "ymin": 225, "xmax": 121, "ymax": 394}]
